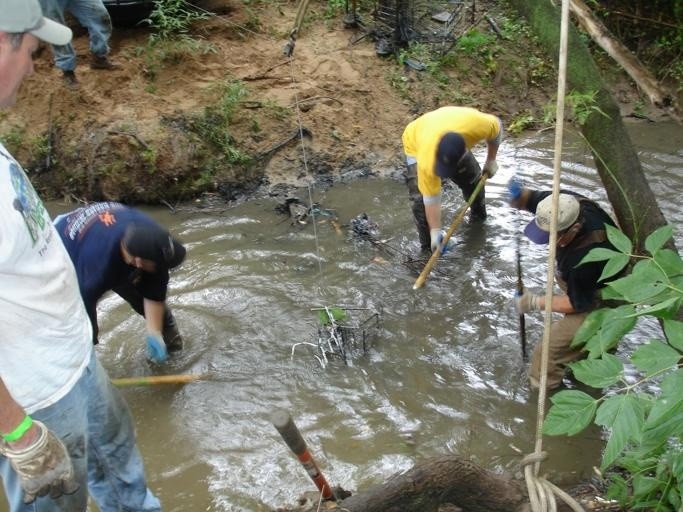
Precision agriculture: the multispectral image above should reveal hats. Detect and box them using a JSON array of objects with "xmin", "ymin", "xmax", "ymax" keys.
[
  {"xmin": 435, "ymin": 133, "xmax": 465, "ymax": 177},
  {"xmin": 0, "ymin": 0, "xmax": 73, "ymax": 46},
  {"xmin": 524, "ymin": 194, "xmax": 580, "ymax": 244},
  {"xmin": 122, "ymin": 222, "xmax": 186, "ymax": 268}
]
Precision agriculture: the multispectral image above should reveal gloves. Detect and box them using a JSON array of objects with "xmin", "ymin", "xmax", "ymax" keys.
[
  {"xmin": 146, "ymin": 335, "xmax": 170, "ymax": 372},
  {"xmin": 430, "ymin": 228, "xmax": 448, "ymax": 255},
  {"xmin": 0, "ymin": 420, "xmax": 81, "ymax": 504},
  {"xmin": 482, "ymin": 160, "xmax": 498, "ymax": 178},
  {"xmin": 513, "ymin": 287, "xmax": 538, "ymax": 314}
]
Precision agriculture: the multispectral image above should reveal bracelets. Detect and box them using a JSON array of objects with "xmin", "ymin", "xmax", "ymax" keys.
[{"xmin": 2, "ymin": 409, "xmax": 35, "ymax": 448}]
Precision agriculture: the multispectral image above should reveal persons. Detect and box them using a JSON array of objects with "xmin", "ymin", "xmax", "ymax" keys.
[
  {"xmin": 506, "ymin": 179, "xmax": 634, "ymax": 399},
  {"xmin": 398, "ymin": 104, "xmax": 502, "ymax": 269},
  {"xmin": 37, "ymin": 1, "xmax": 125, "ymax": 94},
  {"xmin": 43, "ymin": 197, "xmax": 186, "ymax": 368},
  {"xmin": 0, "ymin": 1, "xmax": 165, "ymax": 511}
]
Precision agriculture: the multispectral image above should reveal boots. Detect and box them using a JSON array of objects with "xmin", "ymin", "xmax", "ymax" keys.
[
  {"xmin": 90, "ymin": 56, "xmax": 121, "ymax": 70},
  {"xmin": 64, "ymin": 71, "xmax": 79, "ymax": 88}
]
